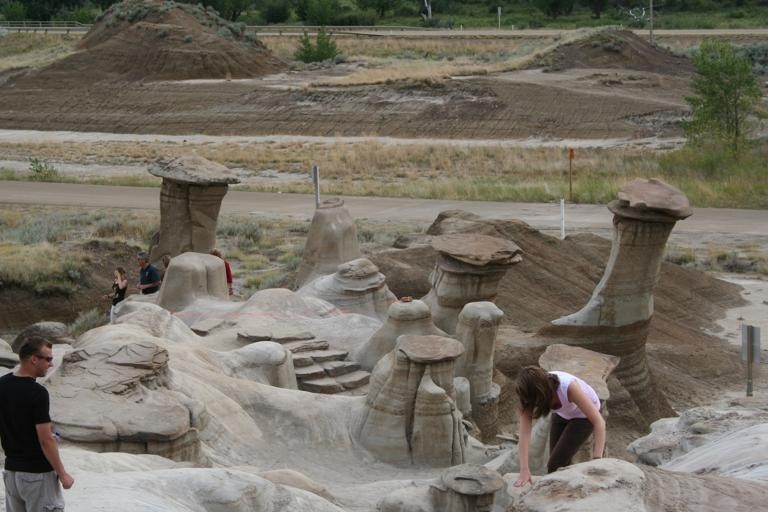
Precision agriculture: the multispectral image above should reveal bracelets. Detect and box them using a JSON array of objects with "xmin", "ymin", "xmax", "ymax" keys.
[{"xmin": 108, "ymin": 295, "xmax": 109, "ymax": 299}]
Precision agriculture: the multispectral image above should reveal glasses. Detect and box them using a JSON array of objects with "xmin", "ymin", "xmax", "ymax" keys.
[{"xmin": 36, "ymin": 355, "xmax": 53, "ymax": 362}]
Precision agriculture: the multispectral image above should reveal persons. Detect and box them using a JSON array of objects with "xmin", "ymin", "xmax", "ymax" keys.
[
  {"xmin": 513, "ymin": 365, "xmax": 606, "ymax": 487},
  {"xmin": 136, "ymin": 250, "xmax": 161, "ymax": 295},
  {"xmin": 0, "ymin": 335, "xmax": 74, "ymax": 512},
  {"xmin": 102, "ymin": 267, "xmax": 128, "ymax": 322},
  {"xmin": 211, "ymin": 247, "xmax": 233, "ymax": 295}
]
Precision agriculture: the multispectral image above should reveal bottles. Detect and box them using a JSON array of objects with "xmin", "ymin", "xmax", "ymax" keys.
[{"xmin": 53, "ymin": 431, "xmax": 60, "ymax": 442}]
